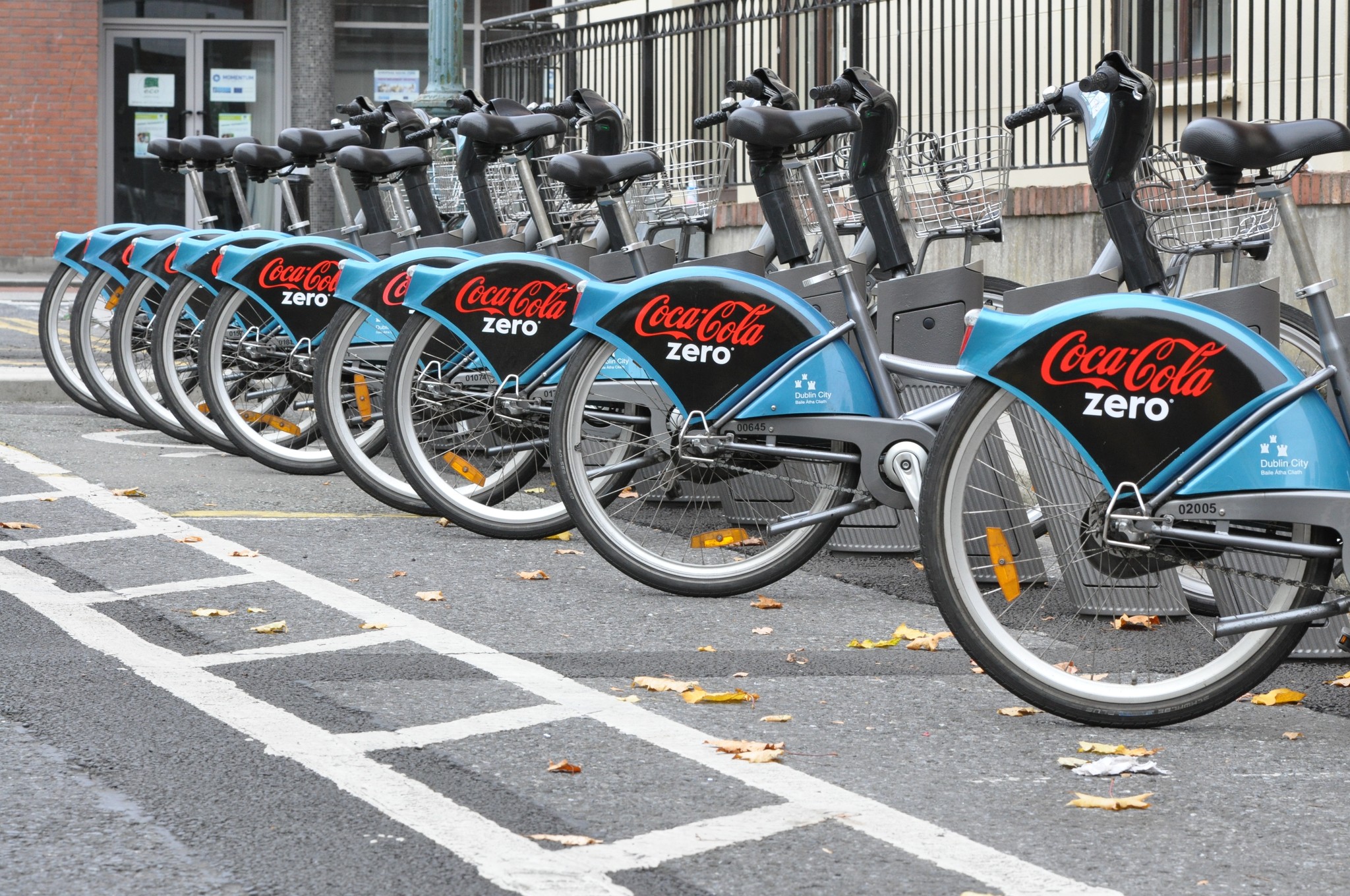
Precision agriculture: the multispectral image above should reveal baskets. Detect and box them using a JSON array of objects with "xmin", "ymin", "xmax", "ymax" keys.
[
  {"xmin": 620, "ymin": 139, "xmax": 734, "ymax": 226},
  {"xmin": 885, "ymin": 124, "xmax": 1014, "ymax": 238},
  {"xmin": 784, "ymin": 147, "xmax": 901, "ymax": 235},
  {"xmin": 1135, "ymin": 151, "xmax": 1289, "ymax": 250},
  {"xmin": 379, "ymin": 146, "xmax": 636, "ymax": 221}
]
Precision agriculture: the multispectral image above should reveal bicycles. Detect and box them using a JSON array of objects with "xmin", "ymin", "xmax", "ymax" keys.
[{"xmin": 37, "ymin": 49, "xmax": 1350, "ymax": 730}]
